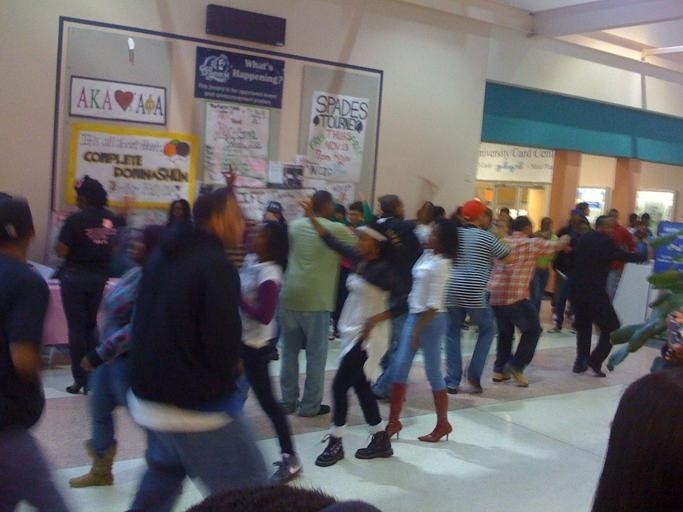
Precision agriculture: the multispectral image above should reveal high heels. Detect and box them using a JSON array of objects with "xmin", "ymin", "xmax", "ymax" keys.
[{"xmin": 66, "ymin": 377, "xmax": 88, "ymax": 395}]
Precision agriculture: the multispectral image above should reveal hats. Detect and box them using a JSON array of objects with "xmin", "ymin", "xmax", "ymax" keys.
[
  {"xmin": 461, "ymin": 199, "xmax": 487, "ymax": 220},
  {"xmin": 266, "ymin": 201, "xmax": 282, "ymax": 213},
  {"xmin": 355, "ymin": 216, "xmax": 392, "ymax": 241}
]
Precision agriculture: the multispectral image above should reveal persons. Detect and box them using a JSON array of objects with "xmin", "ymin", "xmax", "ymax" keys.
[
  {"xmin": 499, "ymin": 207, "xmax": 514, "ymax": 236},
  {"xmin": 164, "ymin": 198, "xmax": 189, "ymax": 232},
  {"xmin": 546, "ymin": 221, "xmax": 591, "ymax": 333},
  {"xmin": 567, "ymin": 215, "xmax": 648, "ymax": 377},
  {"xmin": 70, "ymin": 223, "xmax": 162, "ymax": 488},
  {"xmin": 530, "ymin": 217, "xmax": 572, "ymax": 315},
  {"xmin": 367, "ymin": 195, "xmax": 423, "ymax": 266},
  {"xmin": 625, "ymin": 211, "xmax": 653, "ymax": 242},
  {"xmin": 328, "ymin": 203, "xmax": 357, "ymax": 342},
  {"xmin": 205, "ymin": 189, "xmax": 257, "ymax": 273},
  {"xmin": 557, "ymin": 201, "xmax": 596, "ymax": 237},
  {"xmin": 221, "ymin": 164, "xmax": 302, "ymax": 485},
  {"xmin": 48, "ymin": 176, "xmax": 122, "ymax": 395},
  {"xmin": 274, "ymin": 190, "xmax": 358, "ymax": 418},
  {"xmin": 531, "ymin": 217, "xmax": 571, "ymax": 335},
  {"xmin": 348, "ymin": 191, "xmax": 373, "ymax": 227},
  {"xmin": 486, "ymin": 216, "xmax": 570, "ymax": 387},
  {"xmin": 262, "ymin": 201, "xmax": 286, "ymax": 226},
  {"xmin": 299, "ymin": 193, "xmax": 413, "ymax": 467},
  {"xmin": 605, "ymin": 209, "xmax": 638, "ymax": 306},
  {"xmin": 125, "ymin": 187, "xmax": 268, "ymax": 512},
  {"xmin": 0, "ymin": 198, "xmax": 68, "ymax": 511},
  {"xmin": 442, "ymin": 200, "xmax": 511, "ymax": 394},
  {"xmin": 384, "ymin": 218, "xmax": 459, "ymax": 442}
]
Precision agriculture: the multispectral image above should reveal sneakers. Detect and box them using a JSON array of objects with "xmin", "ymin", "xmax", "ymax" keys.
[
  {"xmin": 573, "ymin": 362, "xmax": 588, "ymax": 373},
  {"xmin": 270, "ymin": 453, "xmax": 302, "ymax": 484},
  {"xmin": 587, "ymin": 360, "xmax": 607, "ymax": 377},
  {"xmin": 546, "ymin": 324, "xmax": 561, "ymax": 333},
  {"xmin": 317, "ymin": 404, "xmax": 330, "ymax": 414},
  {"xmin": 506, "ymin": 363, "xmax": 529, "ymax": 385},
  {"xmin": 492, "ymin": 371, "xmax": 510, "ymax": 382},
  {"xmin": 355, "ymin": 431, "xmax": 393, "ymax": 459},
  {"xmin": 315, "ymin": 434, "xmax": 344, "ymax": 466}
]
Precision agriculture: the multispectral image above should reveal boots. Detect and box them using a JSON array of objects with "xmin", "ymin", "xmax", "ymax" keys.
[
  {"xmin": 385, "ymin": 382, "xmax": 407, "ymax": 440},
  {"xmin": 418, "ymin": 389, "xmax": 453, "ymax": 442},
  {"xmin": 69, "ymin": 439, "xmax": 117, "ymax": 487}
]
